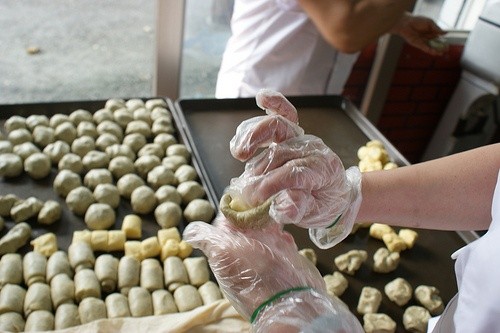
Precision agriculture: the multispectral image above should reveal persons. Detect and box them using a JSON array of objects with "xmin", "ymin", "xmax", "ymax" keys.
[
  {"xmin": 183, "ymin": 90, "xmax": 500, "ymax": 333},
  {"xmin": 215, "ymin": 0, "xmax": 448, "ymax": 99}
]
